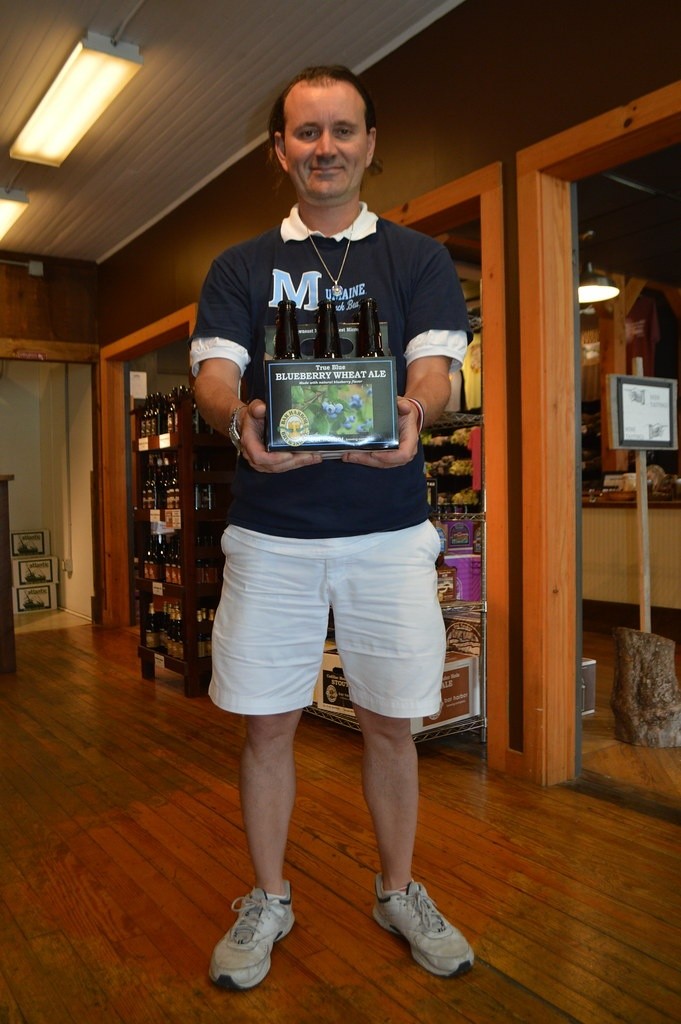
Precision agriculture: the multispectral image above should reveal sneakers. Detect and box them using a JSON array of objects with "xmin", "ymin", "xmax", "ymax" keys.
[
  {"xmin": 208, "ymin": 880, "xmax": 294, "ymax": 990},
  {"xmin": 373, "ymin": 874, "xmax": 473, "ymax": 977}
]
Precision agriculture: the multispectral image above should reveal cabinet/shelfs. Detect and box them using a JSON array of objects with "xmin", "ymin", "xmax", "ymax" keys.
[{"xmin": 128, "ymin": 395, "xmax": 239, "ymax": 698}]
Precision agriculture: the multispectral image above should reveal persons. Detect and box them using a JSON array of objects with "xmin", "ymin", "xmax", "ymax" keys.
[{"xmin": 190, "ymin": 64, "xmax": 474, "ymax": 989}]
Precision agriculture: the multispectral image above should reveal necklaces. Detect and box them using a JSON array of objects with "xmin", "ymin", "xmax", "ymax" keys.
[{"xmin": 307, "ymin": 208, "xmax": 361, "ymax": 296}]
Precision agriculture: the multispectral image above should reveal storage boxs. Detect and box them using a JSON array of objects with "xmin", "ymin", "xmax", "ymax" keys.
[
  {"xmin": 263, "ymin": 322, "xmax": 400, "ymax": 459},
  {"xmin": 10, "ymin": 527, "xmax": 59, "ymax": 614},
  {"xmin": 309, "ymin": 623, "xmax": 479, "ymax": 738}
]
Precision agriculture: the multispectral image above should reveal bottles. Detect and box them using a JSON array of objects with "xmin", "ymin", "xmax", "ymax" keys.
[
  {"xmin": 314, "ymin": 299, "xmax": 342, "ymax": 358},
  {"xmin": 144, "ymin": 603, "xmax": 157, "ymax": 650},
  {"xmin": 143, "ymin": 451, "xmax": 211, "ymax": 514},
  {"xmin": 142, "ymin": 532, "xmax": 219, "ymax": 584},
  {"xmin": 273, "ymin": 300, "xmax": 302, "ymax": 360},
  {"xmin": 141, "ymin": 385, "xmax": 197, "ymax": 438},
  {"xmin": 157, "ymin": 601, "xmax": 214, "ymax": 659},
  {"xmin": 357, "ymin": 297, "xmax": 385, "ymax": 357}
]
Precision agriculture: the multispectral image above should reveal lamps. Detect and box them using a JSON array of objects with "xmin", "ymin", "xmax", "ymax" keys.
[
  {"xmin": 0, "ymin": 33, "xmax": 147, "ymax": 247},
  {"xmin": 577, "ymin": 261, "xmax": 620, "ymax": 303}
]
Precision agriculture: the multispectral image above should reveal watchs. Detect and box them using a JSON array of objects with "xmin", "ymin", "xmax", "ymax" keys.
[{"xmin": 229, "ymin": 405, "xmax": 248, "ymax": 451}]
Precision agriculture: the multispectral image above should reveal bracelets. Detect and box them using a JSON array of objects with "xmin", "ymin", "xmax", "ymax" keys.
[{"xmin": 407, "ymin": 396, "xmax": 425, "ymax": 433}]
